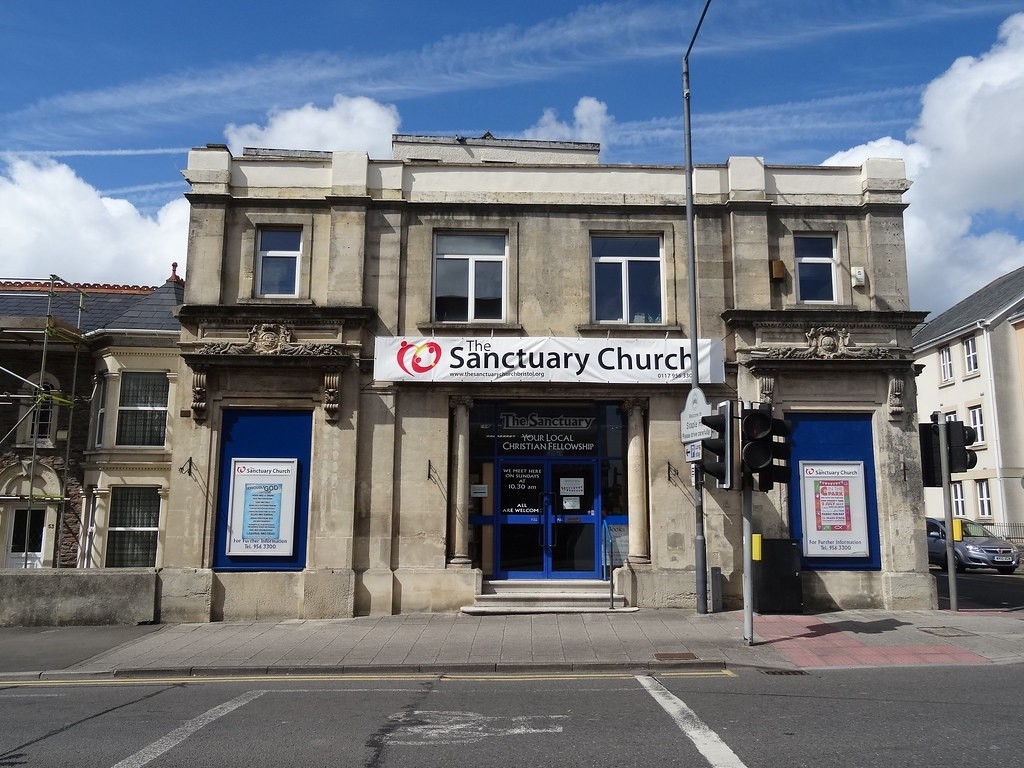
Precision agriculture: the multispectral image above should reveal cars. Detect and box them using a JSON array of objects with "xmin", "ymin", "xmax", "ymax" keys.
[{"xmin": 926, "ymin": 516, "xmax": 1020, "ymax": 574}]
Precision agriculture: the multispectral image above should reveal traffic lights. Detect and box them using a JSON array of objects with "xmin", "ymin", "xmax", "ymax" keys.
[
  {"xmin": 701, "ymin": 400, "xmax": 732, "ymax": 490},
  {"xmin": 948, "ymin": 421, "xmax": 977, "ymax": 472},
  {"xmin": 742, "ymin": 410, "xmax": 791, "ymax": 491}
]
